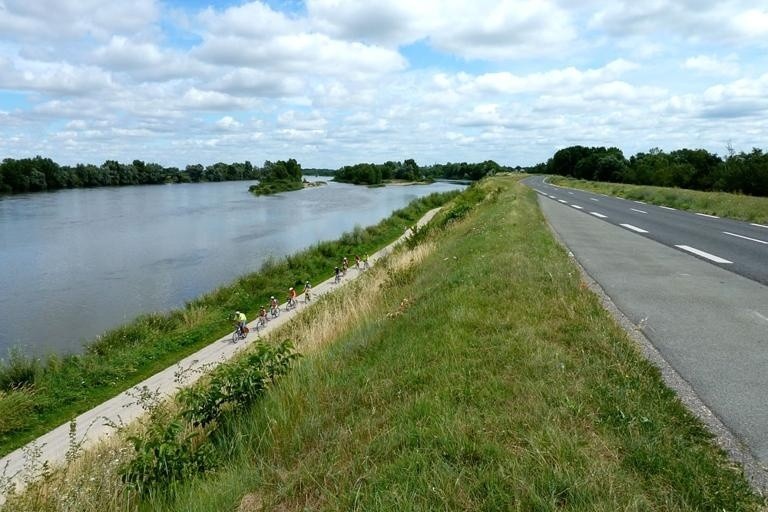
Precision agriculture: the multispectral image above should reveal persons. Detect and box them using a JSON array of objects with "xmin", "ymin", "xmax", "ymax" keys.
[
  {"xmin": 334, "ymin": 265, "xmax": 342, "ymax": 284},
  {"xmin": 361, "ymin": 251, "xmax": 370, "ymax": 270},
  {"xmin": 342, "ymin": 257, "xmax": 349, "ymax": 275},
  {"xmin": 269, "ymin": 296, "xmax": 280, "ymax": 317},
  {"xmin": 289, "ymin": 286, "xmax": 298, "ymax": 304},
  {"xmin": 303, "ymin": 280, "xmax": 312, "ymax": 304},
  {"xmin": 231, "ymin": 311, "xmax": 248, "ymax": 338},
  {"xmin": 354, "ymin": 253, "xmax": 361, "ymax": 270},
  {"xmin": 256, "ymin": 305, "xmax": 271, "ymax": 322}
]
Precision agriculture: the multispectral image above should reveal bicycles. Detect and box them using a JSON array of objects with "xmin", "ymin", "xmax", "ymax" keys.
[{"xmin": 231, "ymin": 259, "xmax": 369, "ymax": 344}]
